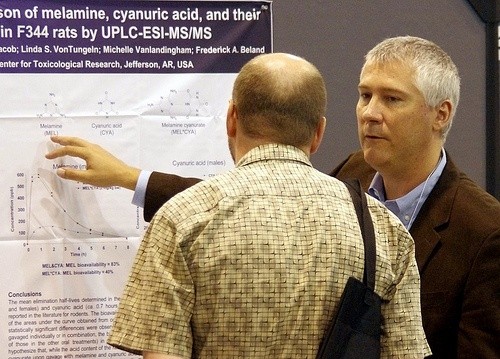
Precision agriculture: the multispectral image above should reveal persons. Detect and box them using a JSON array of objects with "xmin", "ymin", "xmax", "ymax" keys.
[
  {"xmin": 45, "ymin": 35, "xmax": 500, "ymax": 359},
  {"xmin": 105, "ymin": 52, "xmax": 433, "ymax": 359}
]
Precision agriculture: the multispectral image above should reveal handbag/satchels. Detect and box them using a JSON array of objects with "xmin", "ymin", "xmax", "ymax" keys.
[{"xmin": 316, "ymin": 177, "xmax": 391, "ymax": 359}]
